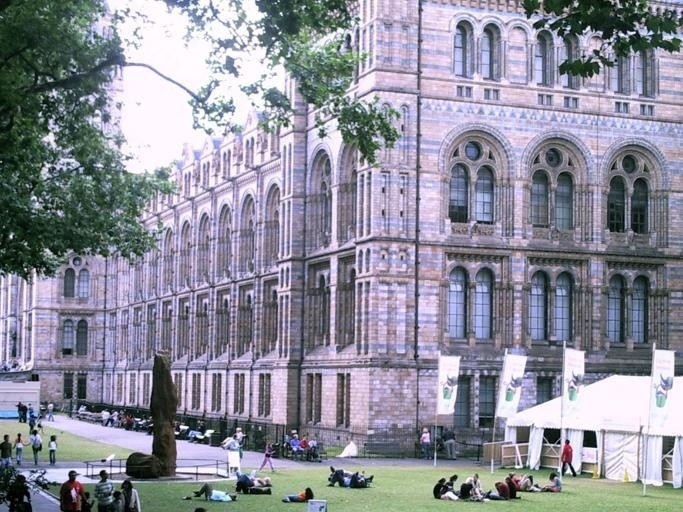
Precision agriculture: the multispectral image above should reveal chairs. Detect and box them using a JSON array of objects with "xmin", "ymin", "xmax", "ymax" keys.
[{"xmin": 54, "ymin": 404, "xmax": 328, "ymax": 461}]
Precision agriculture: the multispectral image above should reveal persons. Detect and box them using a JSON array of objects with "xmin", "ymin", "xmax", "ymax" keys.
[
  {"xmin": 1, "ymin": 429, "xmax": 43, "ymax": 465},
  {"xmin": 6, "ymin": 476, "xmax": 31, "ymax": 511},
  {"xmin": 28, "ymin": 414, "xmax": 44, "ymax": 434},
  {"xmin": 285, "ymin": 434, "xmax": 320, "ymax": 453},
  {"xmin": 234, "ymin": 470, "xmax": 270, "ymax": 494},
  {"xmin": 433, "ymin": 472, "xmax": 562, "ymax": 502},
  {"xmin": 47, "ymin": 436, "xmax": 58, "ymax": 466},
  {"xmin": 222, "ymin": 432, "xmax": 244, "ymax": 473},
  {"xmin": 327, "ymin": 466, "xmax": 373, "ymax": 488},
  {"xmin": 60, "ymin": 470, "xmax": 140, "ymax": 511},
  {"xmin": 16, "ymin": 401, "xmax": 55, "ymax": 423},
  {"xmin": 282, "ymin": 488, "xmax": 314, "ymax": 502},
  {"xmin": 560, "ymin": 440, "xmax": 577, "ymax": 477},
  {"xmin": 415, "ymin": 425, "xmax": 457, "ymax": 460},
  {"xmin": 69, "ymin": 400, "xmax": 252, "ymax": 448},
  {"xmin": 258, "ymin": 440, "xmax": 276, "ymax": 472},
  {"xmin": 193, "ymin": 483, "xmax": 237, "ymax": 501}
]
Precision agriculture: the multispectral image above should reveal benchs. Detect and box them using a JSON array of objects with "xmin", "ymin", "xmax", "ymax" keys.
[{"xmin": 363, "ymin": 441, "xmax": 406, "ymax": 460}]
